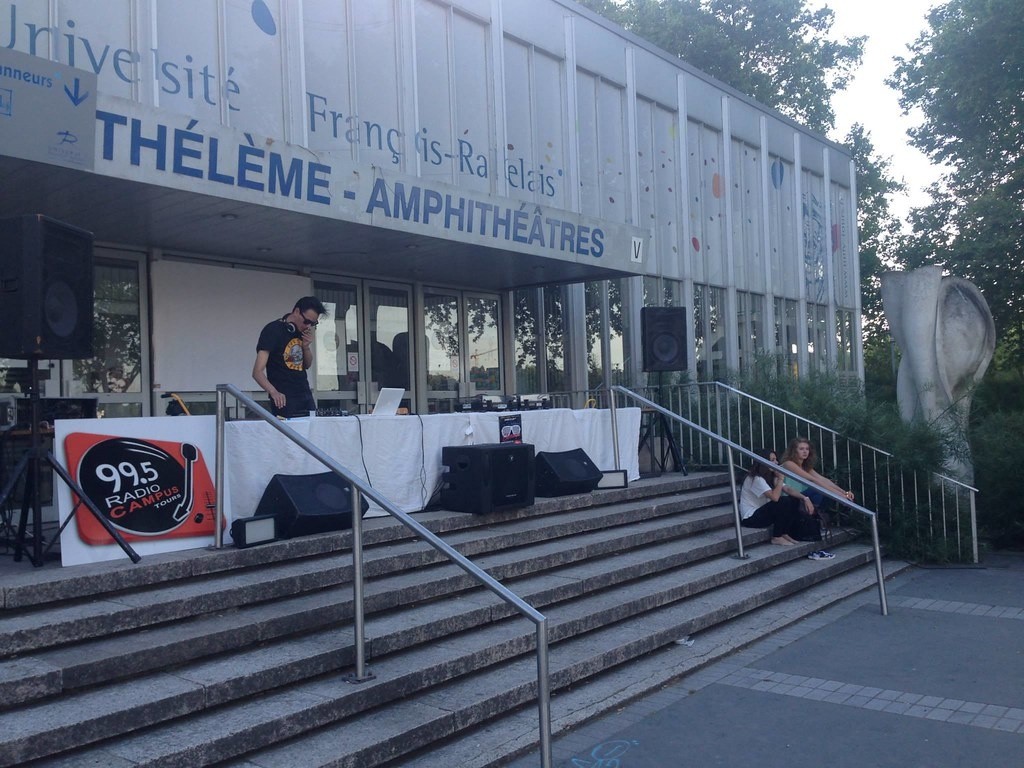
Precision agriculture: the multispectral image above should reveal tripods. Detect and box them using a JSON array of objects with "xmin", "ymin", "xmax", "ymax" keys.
[
  {"xmin": 638, "ymin": 372, "xmax": 689, "ymax": 477},
  {"xmin": 0, "ymin": 359, "xmax": 142, "ymax": 567}
]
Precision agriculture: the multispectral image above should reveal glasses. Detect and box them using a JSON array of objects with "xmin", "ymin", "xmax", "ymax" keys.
[{"xmin": 300, "ymin": 312, "xmax": 318, "ymax": 326}]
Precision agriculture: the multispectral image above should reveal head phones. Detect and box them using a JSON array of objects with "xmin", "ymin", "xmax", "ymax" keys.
[{"xmin": 283, "ymin": 313, "xmax": 296, "ymax": 334}]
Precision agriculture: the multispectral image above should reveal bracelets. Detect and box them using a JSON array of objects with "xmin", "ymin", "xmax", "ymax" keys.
[
  {"xmin": 804, "ymin": 497, "xmax": 809, "ymax": 501},
  {"xmin": 847, "ymin": 495, "xmax": 848, "ymax": 498},
  {"xmin": 846, "ymin": 492, "xmax": 848, "ymax": 494}
]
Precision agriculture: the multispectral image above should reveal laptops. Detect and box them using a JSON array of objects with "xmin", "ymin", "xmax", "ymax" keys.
[{"xmin": 352, "ymin": 388, "xmax": 405, "ymax": 417}]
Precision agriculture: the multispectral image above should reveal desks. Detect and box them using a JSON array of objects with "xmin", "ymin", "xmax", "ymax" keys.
[{"xmin": 0, "ymin": 406, "xmax": 660, "ymax": 569}]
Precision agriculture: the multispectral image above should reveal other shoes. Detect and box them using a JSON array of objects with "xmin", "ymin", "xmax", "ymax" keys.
[{"xmin": 808, "ymin": 550, "xmax": 835, "ymax": 560}]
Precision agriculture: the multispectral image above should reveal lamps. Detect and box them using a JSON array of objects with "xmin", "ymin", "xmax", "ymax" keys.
[
  {"xmin": 595, "ymin": 469, "xmax": 629, "ymax": 491},
  {"xmin": 230, "ymin": 513, "xmax": 277, "ymax": 547}
]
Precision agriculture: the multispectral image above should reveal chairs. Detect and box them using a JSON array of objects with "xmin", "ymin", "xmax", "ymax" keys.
[{"xmin": 15, "ymin": 395, "xmax": 101, "ymax": 547}]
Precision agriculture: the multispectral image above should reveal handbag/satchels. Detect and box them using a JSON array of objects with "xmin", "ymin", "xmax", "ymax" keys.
[{"xmin": 789, "ymin": 510, "xmax": 823, "ymax": 542}]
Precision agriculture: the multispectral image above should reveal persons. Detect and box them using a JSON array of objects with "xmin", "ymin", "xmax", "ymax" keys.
[
  {"xmin": 252, "ymin": 296, "xmax": 325, "ymax": 418},
  {"xmin": 781, "ymin": 438, "xmax": 854, "ymax": 510},
  {"xmin": 740, "ymin": 449, "xmax": 813, "ymax": 546}
]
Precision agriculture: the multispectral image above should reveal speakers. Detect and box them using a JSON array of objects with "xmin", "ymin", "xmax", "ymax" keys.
[
  {"xmin": 232, "ymin": 513, "xmax": 279, "ymax": 548},
  {"xmin": 0, "ymin": 214, "xmax": 95, "ymax": 360},
  {"xmin": 641, "ymin": 305, "xmax": 688, "ymax": 373},
  {"xmin": 440, "ymin": 442, "xmax": 535, "ymax": 514},
  {"xmin": 534, "ymin": 448, "xmax": 603, "ymax": 497},
  {"xmin": 255, "ymin": 471, "xmax": 370, "ymax": 539}
]
